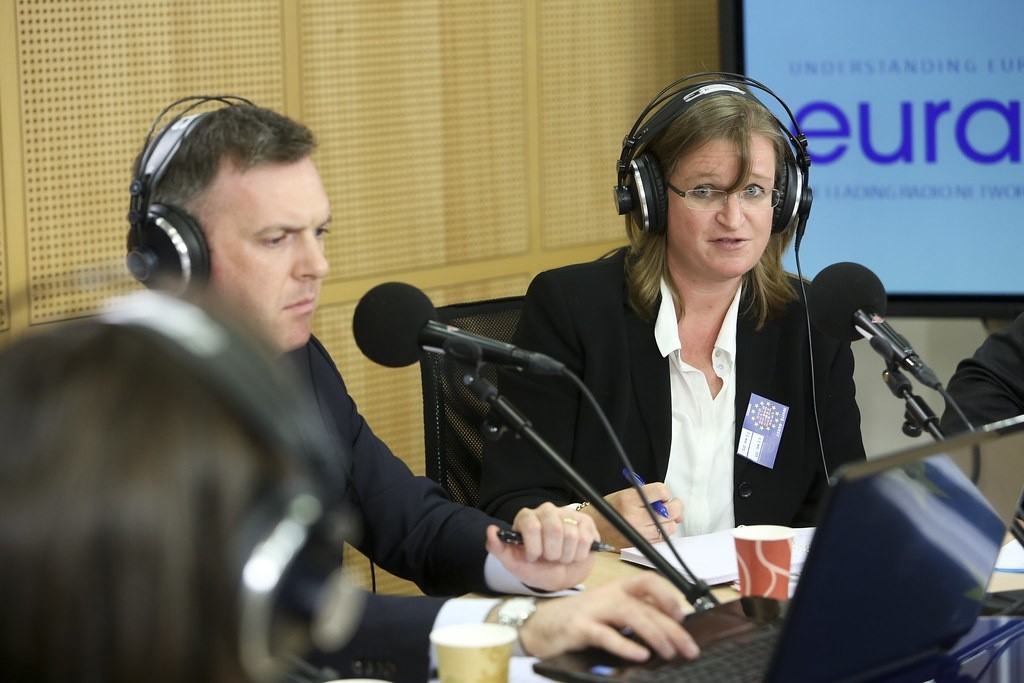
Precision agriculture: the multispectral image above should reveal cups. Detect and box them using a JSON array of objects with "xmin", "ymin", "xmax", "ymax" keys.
[
  {"xmin": 732, "ymin": 523, "xmax": 792, "ymax": 604},
  {"xmin": 428, "ymin": 620, "xmax": 519, "ymax": 683}
]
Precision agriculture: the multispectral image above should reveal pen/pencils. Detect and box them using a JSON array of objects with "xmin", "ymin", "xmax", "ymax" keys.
[
  {"xmin": 497, "ymin": 529, "xmax": 621, "ymax": 554},
  {"xmin": 621, "ymin": 468, "xmax": 670, "ymax": 519}
]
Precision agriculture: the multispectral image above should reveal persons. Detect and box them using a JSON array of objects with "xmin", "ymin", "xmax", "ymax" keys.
[
  {"xmin": 0, "ymin": 282, "xmax": 359, "ymax": 683},
  {"xmin": 123, "ymin": 95, "xmax": 697, "ymax": 683},
  {"xmin": 475, "ymin": 72, "xmax": 866, "ymax": 547},
  {"xmin": 938, "ymin": 310, "xmax": 1024, "ymax": 437}
]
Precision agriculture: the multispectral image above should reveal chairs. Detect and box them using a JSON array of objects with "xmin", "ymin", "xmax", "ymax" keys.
[{"xmin": 422, "ymin": 293, "xmax": 526, "ymax": 504}]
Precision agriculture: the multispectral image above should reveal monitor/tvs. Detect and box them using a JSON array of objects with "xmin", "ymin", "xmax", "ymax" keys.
[{"xmin": 718, "ymin": 0, "xmax": 1024, "ymax": 321}]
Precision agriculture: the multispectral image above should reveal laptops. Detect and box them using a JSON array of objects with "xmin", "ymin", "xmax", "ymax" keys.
[{"xmin": 533, "ymin": 415, "xmax": 1024, "ymax": 683}]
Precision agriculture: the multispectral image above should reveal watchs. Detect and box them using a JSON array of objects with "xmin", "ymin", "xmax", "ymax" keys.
[{"xmin": 498, "ymin": 596, "xmax": 536, "ymax": 656}]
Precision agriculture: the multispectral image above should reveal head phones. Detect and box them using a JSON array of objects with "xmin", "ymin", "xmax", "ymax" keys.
[
  {"xmin": 126, "ymin": 96, "xmax": 258, "ymax": 299},
  {"xmin": 103, "ymin": 289, "xmax": 365, "ymax": 683},
  {"xmin": 613, "ymin": 71, "xmax": 813, "ymax": 234}
]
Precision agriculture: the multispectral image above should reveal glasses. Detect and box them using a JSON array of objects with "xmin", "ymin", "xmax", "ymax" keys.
[{"xmin": 667, "ymin": 182, "xmax": 781, "ymax": 211}]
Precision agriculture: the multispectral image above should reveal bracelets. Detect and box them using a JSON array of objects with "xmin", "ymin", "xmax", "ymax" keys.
[{"xmin": 577, "ymin": 501, "xmax": 593, "ymax": 512}]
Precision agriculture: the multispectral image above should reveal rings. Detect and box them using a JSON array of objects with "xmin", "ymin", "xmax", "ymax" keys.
[
  {"xmin": 656, "ymin": 529, "xmax": 665, "ymax": 544},
  {"xmin": 562, "ymin": 516, "xmax": 577, "ymax": 523}
]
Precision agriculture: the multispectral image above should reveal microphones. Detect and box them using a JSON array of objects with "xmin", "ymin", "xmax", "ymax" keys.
[
  {"xmin": 806, "ymin": 262, "xmax": 942, "ymax": 391},
  {"xmin": 353, "ymin": 282, "xmax": 564, "ymax": 376}
]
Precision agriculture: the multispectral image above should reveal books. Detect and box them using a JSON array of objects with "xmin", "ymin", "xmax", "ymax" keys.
[{"xmin": 620, "ymin": 523, "xmax": 816, "ymax": 587}]
